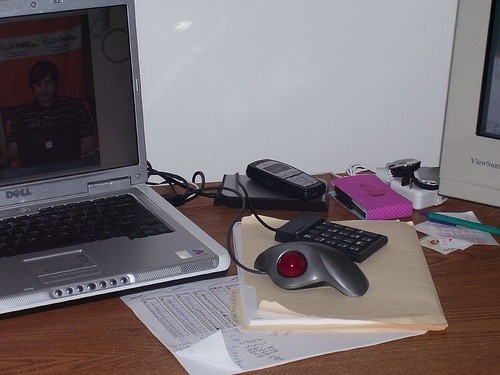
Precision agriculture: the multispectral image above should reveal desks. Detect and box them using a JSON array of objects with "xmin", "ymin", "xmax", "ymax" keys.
[{"xmin": 0, "ymin": 165, "xmax": 499, "ymax": 375}]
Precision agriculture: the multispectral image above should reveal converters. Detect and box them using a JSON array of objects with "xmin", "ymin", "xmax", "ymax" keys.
[{"xmin": 388, "ymin": 177, "xmax": 443, "ymax": 210}]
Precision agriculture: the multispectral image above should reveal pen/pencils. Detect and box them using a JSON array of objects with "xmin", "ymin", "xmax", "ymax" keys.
[{"xmin": 420, "ymin": 210, "xmax": 500, "ymax": 235}]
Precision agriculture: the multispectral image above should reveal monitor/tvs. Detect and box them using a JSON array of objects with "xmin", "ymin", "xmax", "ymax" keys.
[{"xmin": 438, "ymin": 1, "xmax": 500, "ymax": 206}]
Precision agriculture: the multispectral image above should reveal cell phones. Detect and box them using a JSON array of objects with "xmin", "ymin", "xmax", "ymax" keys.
[{"xmin": 246, "ymin": 157, "xmax": 326, "ymax": 200}]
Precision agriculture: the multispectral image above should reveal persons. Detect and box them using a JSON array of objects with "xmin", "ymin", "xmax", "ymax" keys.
[{"xmin": 9, "ymin": 60, "xmax": 97, "ymax": 167}]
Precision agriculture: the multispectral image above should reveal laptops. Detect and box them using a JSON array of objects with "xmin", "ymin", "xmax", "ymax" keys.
[{"xmin": 1, "ymin": 0, "xmax": 231, "ymax": 314}]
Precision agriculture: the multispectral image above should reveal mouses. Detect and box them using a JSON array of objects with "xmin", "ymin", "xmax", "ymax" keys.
[{"xmin": 253, "ymin": 240, "xmax": 369, "ymax": 299}]
